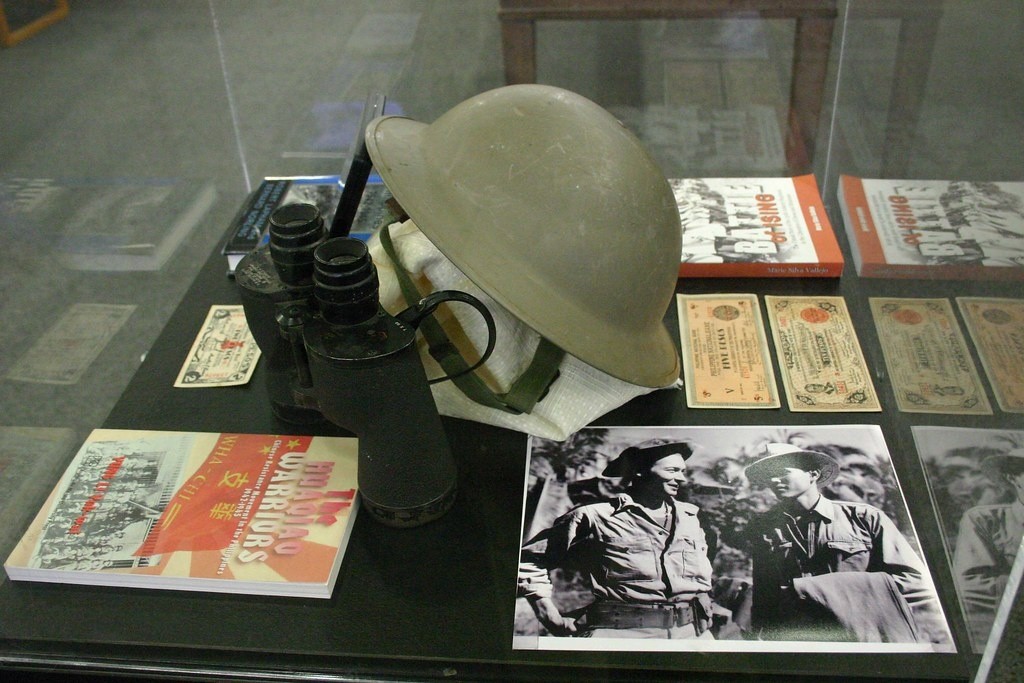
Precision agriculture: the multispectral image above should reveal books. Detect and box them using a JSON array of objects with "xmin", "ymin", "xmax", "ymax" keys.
[
  {"xmin": 838, "ymin": 163, "xmax": 1022, "ymax": 277},
  {"xmin": 212, "ymin": 178, "xmax": 418, "ymax": 279},
  {"xmin": 3, "ymin": 426, "xmax": 362, "ymax": 600},
  {"xmin": 667, "ymin": 174, "xmax": 846, "ymax": 277}
]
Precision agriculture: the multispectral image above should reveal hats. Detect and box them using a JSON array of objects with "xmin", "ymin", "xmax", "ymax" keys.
[
  {"xmin": 986, "ymin": 449, "xmax": 1024, "ymax": 467},
  {"xmin": 602, "ymin": 438, "xmax": 695, "ymax": 477},
  {"xmin": 744, "ymin": 443, "xmax": 841, "ymax": 489}
]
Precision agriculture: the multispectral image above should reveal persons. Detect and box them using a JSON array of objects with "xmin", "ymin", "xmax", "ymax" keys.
[
  {"xmin": 951, "ymin": 449, "xmax": 1023, "ymax": 653},
  {"xmin": 745, "ymin": 443, "xmax": 949, "ymax": 644},
  {"xmin": 544, "ymin": 438, "xmax": 720, "ymax": 638}
]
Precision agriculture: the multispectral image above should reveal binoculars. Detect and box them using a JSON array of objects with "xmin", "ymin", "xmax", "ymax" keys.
[{"xmin": 233, "ymin": 203, "xmax": 459, "ymax": 527}]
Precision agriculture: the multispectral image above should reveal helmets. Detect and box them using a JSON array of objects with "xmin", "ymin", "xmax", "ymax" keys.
[{"xmin": 364, "ymin": 84, "xmax": 683, "ymax": 388}]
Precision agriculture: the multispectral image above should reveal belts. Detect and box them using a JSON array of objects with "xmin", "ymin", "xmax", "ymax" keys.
[{"xmin": 587, "ymin": 602, "xmax": 699, "ymax": 630}]
